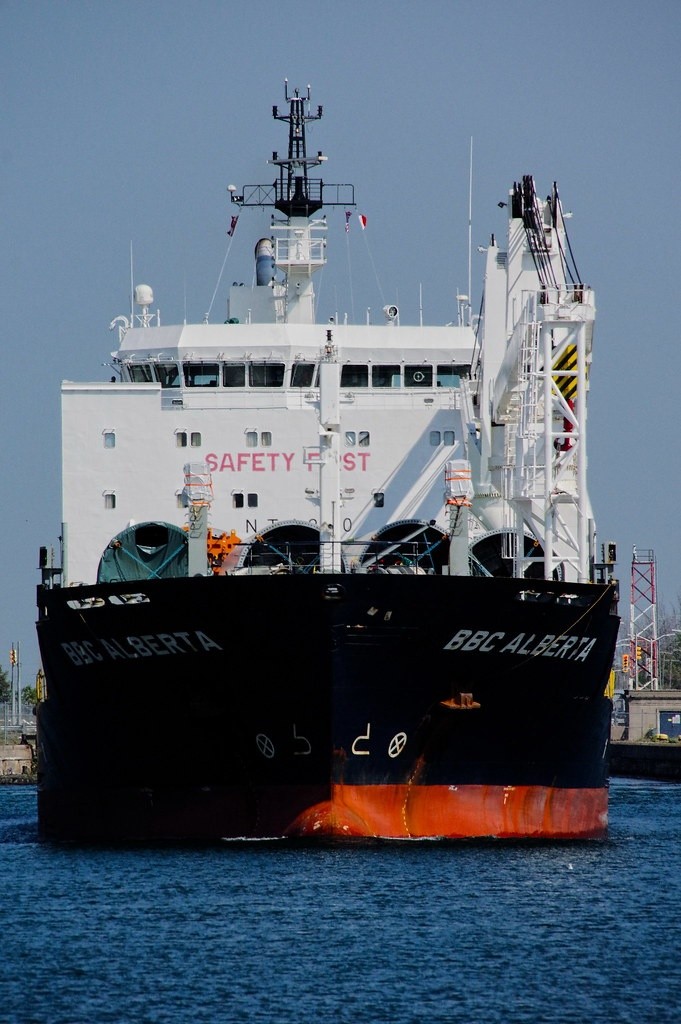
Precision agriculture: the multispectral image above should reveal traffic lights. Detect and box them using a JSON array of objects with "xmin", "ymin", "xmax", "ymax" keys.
[
  {"xmin": 10, "ymin": 650, "xmax": 14, "ymax": 664},
  {"xmin": 637, "ymin": 647, "xmax": 641, "ymax": 660},
  {"xmin": 624, "ymin": 655, "xmax": 628, "ymax": 671}
]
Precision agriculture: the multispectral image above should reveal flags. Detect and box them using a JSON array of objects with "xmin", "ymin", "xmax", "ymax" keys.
[
  {"xmin": 359, "ymin": 213, "xmax": 366, "ymax": 229},
  {"xmin": 226, "ymin": 216, "xmax": 237, "ymax": 237},
  {"xmin": 345, "ymin": 211, "xmax": 352, "ymax": 232}
]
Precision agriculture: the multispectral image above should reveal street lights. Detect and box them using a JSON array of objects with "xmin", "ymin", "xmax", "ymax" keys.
[{"xmin": 627, "ymin": 633, "xmax": 676, "ymax": 689}]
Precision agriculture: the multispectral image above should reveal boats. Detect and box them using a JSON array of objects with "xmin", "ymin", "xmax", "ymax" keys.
[{"xmin": 20, "ymin": 78, "xmax": 623, "ymax": 846}]
